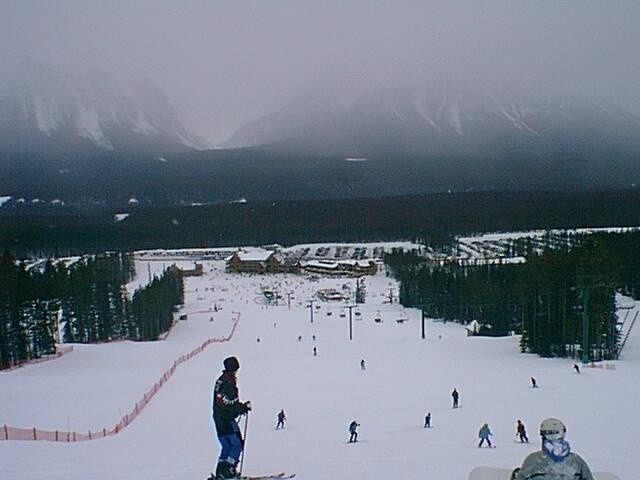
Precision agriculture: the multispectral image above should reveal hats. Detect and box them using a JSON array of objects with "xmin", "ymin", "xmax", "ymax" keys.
[{"xmin": 224, "ymin": 357, "xmax": 240, "ymax": 371}]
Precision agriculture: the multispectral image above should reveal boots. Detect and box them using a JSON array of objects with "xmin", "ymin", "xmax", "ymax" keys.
[{"xmin": 216, "ymin": 461, "xmax": 238, "ymax": 478}]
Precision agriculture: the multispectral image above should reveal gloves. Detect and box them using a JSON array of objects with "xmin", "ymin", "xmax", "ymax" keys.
[{"xmin": 240, "ymin": 401, "xmax": 251, "ymax": 416}]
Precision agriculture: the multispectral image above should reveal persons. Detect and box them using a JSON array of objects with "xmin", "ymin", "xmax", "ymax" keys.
[
  {"xmin": 313, "ymin": 347, "xmax": 316, "ymax": 356},
  {"xmin": 349, "ymin": 422, "xmax": 360, "ymax": 443},
  {"xmin": 212, "ymin": 357, "xmax": 252, "ymax": 480},
  {"xmin": 516, "ymin": 420, "xmax": 528, "ymax": 443},
  {"xmin": 509, "ymin": 418, "xmax": 595, "ymax": 480},
  {"xmin": 312, "ymin": 335, "xmax": 315, "ymax": 341},
  {"xmin": 424, "ymin": 414, "xmax": 431, "ymax": 428},
  {"xmin": 361, "ymin": 360, "xmax": 366, "ymax": 370},
  {"xmin": 574, "ymin": 365, "xmax": 580, "ymax": 374},
  {"xmin": 299, "ymin": 336, "xmax": 301, "ymax": 342},
  {"xmin": 531, "ymin": 376, "xmax": 536, "ymax": 388},
  {"xmin": 452, "ymin": 389, "xmax": 459, "ymax": 408},
  {"xmin": 276, "ymin": 411, "xmax": 286, "ymax": 430},
  {"xmin": 478, "ymin": 424, "xmax": 492, "ymax": 448}
]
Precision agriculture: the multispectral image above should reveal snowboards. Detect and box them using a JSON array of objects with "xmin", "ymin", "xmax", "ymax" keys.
[{"xmin": 470, "ymin": 466, "xmax": 621, "ymax": 479}]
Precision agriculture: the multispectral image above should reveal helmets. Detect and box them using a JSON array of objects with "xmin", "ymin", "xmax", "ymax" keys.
[{"xmin": 540, "ymin": 418, "xmax": 566, "ymax": 442}]
